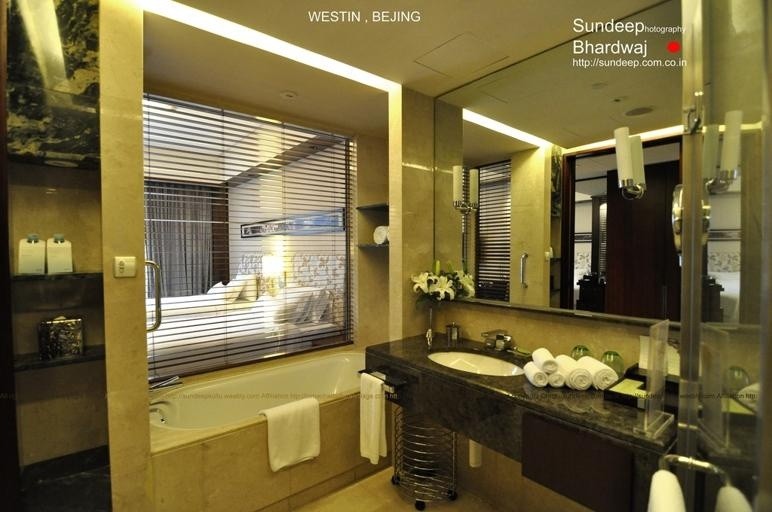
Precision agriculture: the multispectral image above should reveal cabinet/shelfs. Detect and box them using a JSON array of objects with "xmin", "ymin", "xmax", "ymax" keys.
[
  {"xmin": 356, "ymin": 201, "xmax": 389, "ymax": 248},
  {"xmin": 550, "ymin": 257, "xmax": 562, "ymax": 291},
  {"xmin": 12, "ymin": 272, "xmax": 106, "ymax": 373}
]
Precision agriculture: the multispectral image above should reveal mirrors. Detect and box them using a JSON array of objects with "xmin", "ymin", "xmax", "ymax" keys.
[{"xmin": 432, "ymin": 0, "xmax": 772, "ymax": 330}]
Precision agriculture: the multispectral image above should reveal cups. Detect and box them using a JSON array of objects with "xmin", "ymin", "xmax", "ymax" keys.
[
  {"xmin": 571, "ymin": 344, "xmax": 594, "ymax": 361},
  {"xmin": 600, "ymin": 350, "xmax": 625, "ymax": 379}
]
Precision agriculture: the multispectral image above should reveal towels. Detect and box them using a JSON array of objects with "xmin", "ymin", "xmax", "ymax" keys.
[
  {"xmin": 646, "ymin": 469, "xmax": 685, "ymax": 512},
  {"xmin": 359, "ymin": 372, "xmax": 387, "ymax": 465},
  {"xmin": 715, "ymin": 485, "xmax": 754, "ymax": 512},
  {"xmin": 373, "ymin": 225, "xmax": 390, "ymax": 245},
  {"xmin": 523, "ymin": 347, "xmax": 619, "ymax": 390},
  {"xmin": 259, "ymin": 397, "xmax": 321, "ymax": 473}
]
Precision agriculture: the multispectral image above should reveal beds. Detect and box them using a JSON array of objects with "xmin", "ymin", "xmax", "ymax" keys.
[{"xmin": 145, "ymin": 252, "xmax": 347, "ymax": 380}]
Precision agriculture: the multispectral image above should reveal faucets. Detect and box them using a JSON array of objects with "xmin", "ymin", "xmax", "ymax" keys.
[
  {"xmin": 478, "ymin": 340, "xmax": 495, "ymax": 353},
  {"xmin": 149, "ymin": 407, "xmax": 168, "ymax": 424}
]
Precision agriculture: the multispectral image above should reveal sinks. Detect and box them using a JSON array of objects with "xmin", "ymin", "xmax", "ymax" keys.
[{"xmin": 426, "ymin": 347, "xmax": 525, "ymax": 376}]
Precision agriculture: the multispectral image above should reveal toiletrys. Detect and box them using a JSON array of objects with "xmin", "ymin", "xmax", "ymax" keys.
[{"xmin": 625, "ymin": 333, "xmax": 702, "ymax": 392}]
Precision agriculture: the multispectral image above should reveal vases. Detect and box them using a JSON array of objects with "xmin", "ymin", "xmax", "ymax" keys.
[{"xmin": 424, "ymin": 301, "xmax": 435, "ymax": 338}]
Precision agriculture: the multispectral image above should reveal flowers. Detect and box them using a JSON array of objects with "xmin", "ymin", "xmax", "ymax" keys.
[
  {"xmin": 441, "ymin": 260, "xmax": 476, "ymax": 299},
  {"xmin": 410, "ymin": 260, "xmax": 455, "ymax": 311}
]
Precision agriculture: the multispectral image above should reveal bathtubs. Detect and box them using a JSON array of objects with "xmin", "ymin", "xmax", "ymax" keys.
[{"xmin": 150, "ymin": 352, "xmax": 365, "ymax": 431}]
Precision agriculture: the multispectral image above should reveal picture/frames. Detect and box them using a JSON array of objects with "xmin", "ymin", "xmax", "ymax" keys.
[{"xmin": 241, "ymin": 207, "xmax": 345, "ymax": 238}]
